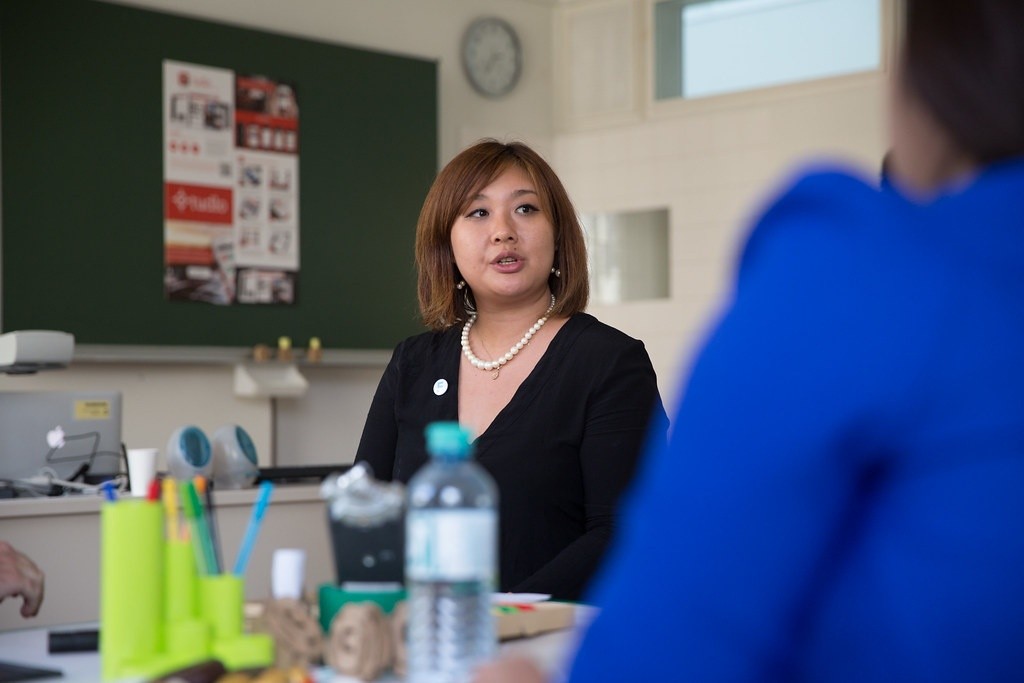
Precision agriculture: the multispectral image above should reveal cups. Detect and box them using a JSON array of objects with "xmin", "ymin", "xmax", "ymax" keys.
[
  {"xmin": 199, "ymin": 575, "xmax": 243, "ymax": 638},
  {"xmin": 126, "ymin": 448, "xmax": 156, "ymax": 494}
]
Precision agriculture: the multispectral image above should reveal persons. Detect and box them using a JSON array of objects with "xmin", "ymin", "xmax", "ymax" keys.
[
  {"xmin": 329, "ymin": 138, "xmax": 670, "ymax": 605},
  {"xmin": 560, "ymin": 1, "xmax": 1024, "ymax": 683},
  {"xmin": 0, "ymin": 539, "xmax": 46, "ymax": 617}
]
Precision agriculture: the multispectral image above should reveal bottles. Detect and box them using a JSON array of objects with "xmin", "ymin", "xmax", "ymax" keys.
[{"xmin": 404, "ymin": 422, "xmax": 498, "ymax": 683}]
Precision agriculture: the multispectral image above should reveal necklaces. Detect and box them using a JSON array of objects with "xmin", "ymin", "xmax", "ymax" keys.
[{"xmin": 461, "ymin": 293, "xmax": 555, "ymax": 380}]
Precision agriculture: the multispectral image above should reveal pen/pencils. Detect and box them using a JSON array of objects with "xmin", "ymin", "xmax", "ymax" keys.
[{"xmin": 100, "ymin": 470, "xmax": 276, "ymax": 575}]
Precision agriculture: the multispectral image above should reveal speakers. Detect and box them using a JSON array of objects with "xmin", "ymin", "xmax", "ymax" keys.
[
  {"xmin": 166, "ymin": 426, "xmax": 213, "ymax": 488},
  {"xmin": 210, "ymin": 424, "xmax": 259, "ymax": 491}
]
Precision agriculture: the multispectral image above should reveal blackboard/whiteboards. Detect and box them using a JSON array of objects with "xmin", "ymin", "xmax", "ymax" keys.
[{"xmin": 0, "ymin": 0, "xmax": 444, "ymax": 369}]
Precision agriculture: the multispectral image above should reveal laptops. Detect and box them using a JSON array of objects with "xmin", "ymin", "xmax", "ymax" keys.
[{"xmin": 0, "ymin": 390, "xmax": 124, "ymax": 496}]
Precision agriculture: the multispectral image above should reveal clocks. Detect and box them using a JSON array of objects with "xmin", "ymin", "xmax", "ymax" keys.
[{"xmin": 459, "ymin": 14, "xmax": 522, "ymax": 99}]
